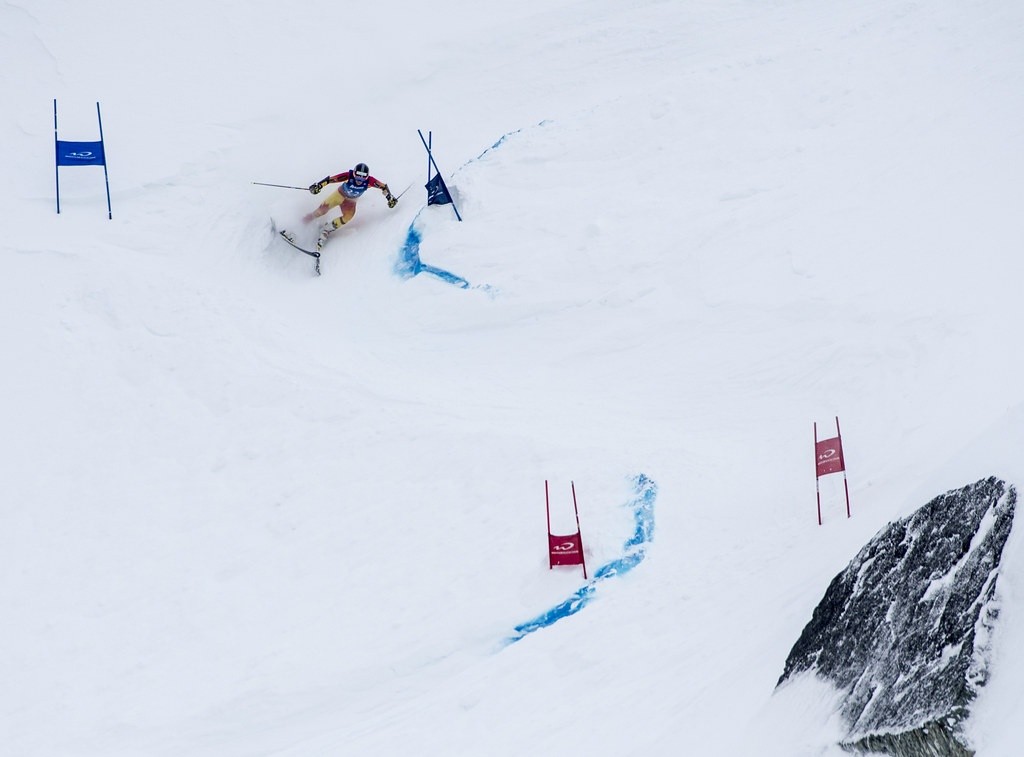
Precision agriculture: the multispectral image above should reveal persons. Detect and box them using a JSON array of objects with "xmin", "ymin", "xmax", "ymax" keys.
[{"xmin": 300, "ymin": 162, "xmax": 397, "ymax": 241}]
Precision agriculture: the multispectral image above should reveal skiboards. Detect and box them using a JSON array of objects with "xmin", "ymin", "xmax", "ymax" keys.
[{"xmin": 272, "ymin": 219, "xmax": 327, "ymax": 276}]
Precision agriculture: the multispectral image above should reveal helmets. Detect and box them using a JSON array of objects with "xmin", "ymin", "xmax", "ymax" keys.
[{"xmin": 353, "ymin": 163, "xmax": 369, "ymax": 181}]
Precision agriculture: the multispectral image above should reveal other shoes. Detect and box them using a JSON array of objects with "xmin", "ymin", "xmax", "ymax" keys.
[{"xmin": 321, "ymin": 225, "xmax": 329, "ymax": 240}]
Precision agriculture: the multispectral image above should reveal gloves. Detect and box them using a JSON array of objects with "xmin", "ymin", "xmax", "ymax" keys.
[
  {"xmin": 309, "ymin": 183, "xmax": 321, "ymax": 195},
  {"xmin": 386, "ymin": 194, "xmax": 397, "ymax": 208}
]
